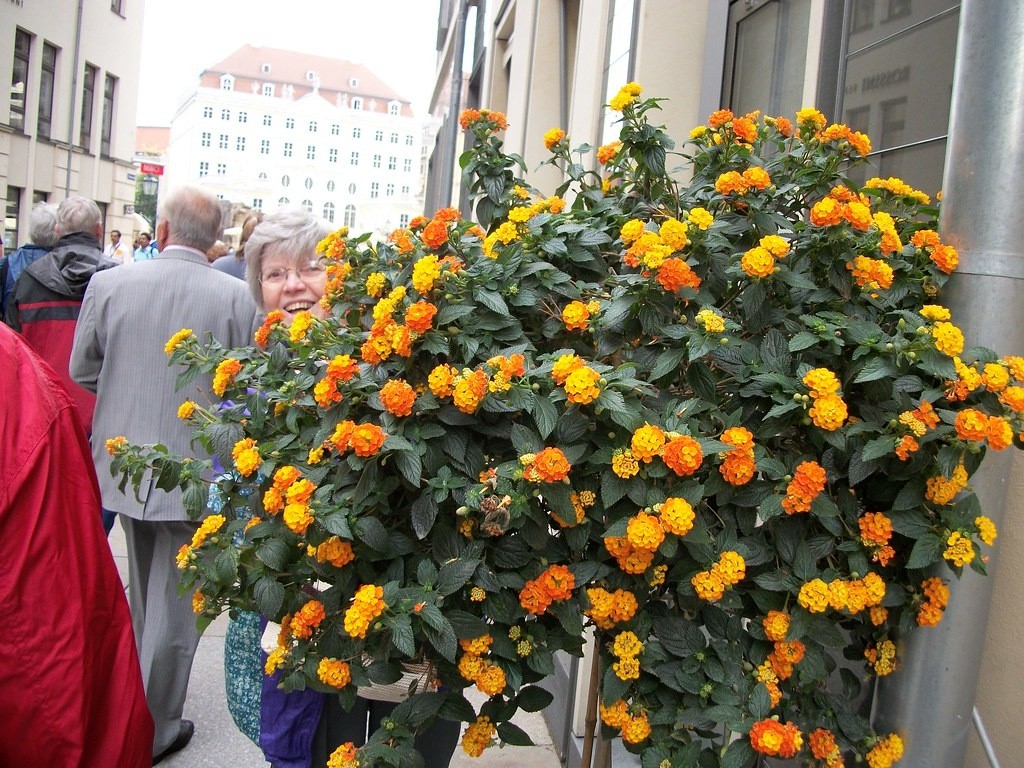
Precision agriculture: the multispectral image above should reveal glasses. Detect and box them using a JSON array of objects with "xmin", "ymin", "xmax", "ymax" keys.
[{"xmin": 259, "ymin": 260, "xmax": 330, "ymax": 286}]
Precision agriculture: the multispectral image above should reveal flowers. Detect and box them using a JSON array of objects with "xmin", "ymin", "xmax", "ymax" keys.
[{"xmin": 82, "ymin": 79, "xmax": 1023, "ymax": 767}]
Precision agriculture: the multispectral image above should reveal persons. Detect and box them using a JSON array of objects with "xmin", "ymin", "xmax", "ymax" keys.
[{"xmin": 0, "ymin": 181, "xmax": 465, "ymax": 767}]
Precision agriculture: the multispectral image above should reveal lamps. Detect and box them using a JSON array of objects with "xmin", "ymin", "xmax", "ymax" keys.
[{"xmin": 142, "ymin": 173, "xmax": 159, "ymax": 201}]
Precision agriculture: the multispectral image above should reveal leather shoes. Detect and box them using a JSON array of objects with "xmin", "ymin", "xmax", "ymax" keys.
[{"xmin": 153, "ymin": 717, "xmax": 195, "ymax": 767}]
